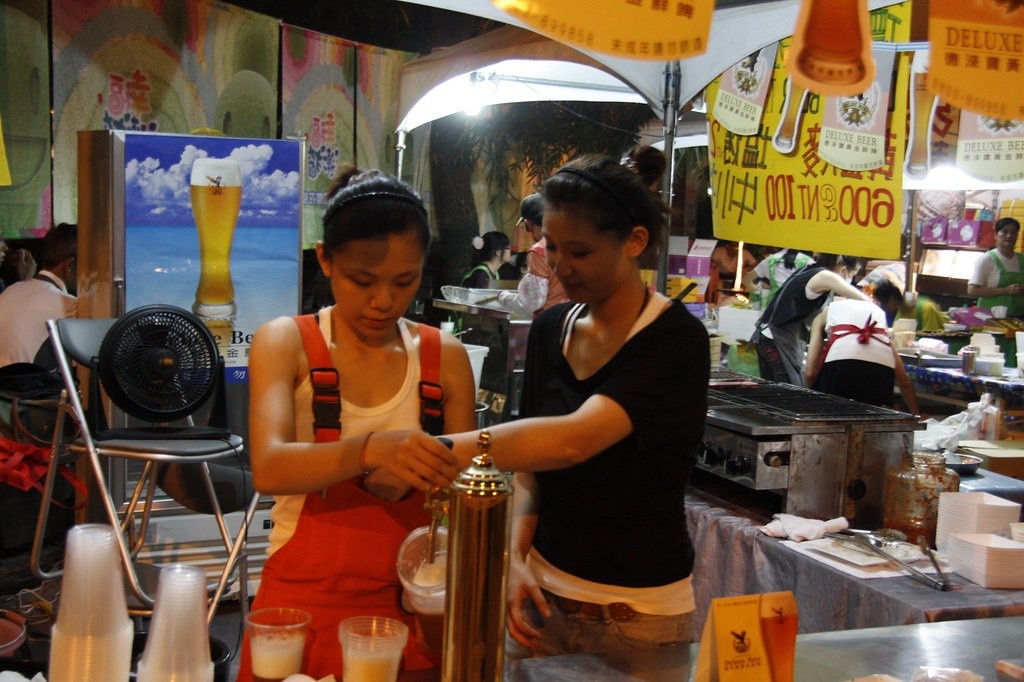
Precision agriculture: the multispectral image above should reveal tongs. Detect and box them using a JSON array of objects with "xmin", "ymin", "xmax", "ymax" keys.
[
  {"xmin": 854, "ymin": 533, "xmax": 948, "ymax": 592},
  {"xmin": 823, "ymin": 529, "xmax": 908, "ymax": 551}
]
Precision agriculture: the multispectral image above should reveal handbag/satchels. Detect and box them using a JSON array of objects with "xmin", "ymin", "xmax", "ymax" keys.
[
  {"xmin": 0, "ymin": 433, "xmax": 87, "ymax": 509},
  {"xmin": 952, "ymin": 306, "xmax": 995, "ymax": 327},
  {"xmin": 913, "ymin": 409, "xmax": 969, "ymax": 453},
  {"xmin": 727, "ymin": 342, "xmax": 761, "ymax": 377}
]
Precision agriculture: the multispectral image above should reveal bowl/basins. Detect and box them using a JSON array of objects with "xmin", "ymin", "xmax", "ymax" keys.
[{"xmin": 945, "ymin": 454, "xmax": 985, "ymax": 475}]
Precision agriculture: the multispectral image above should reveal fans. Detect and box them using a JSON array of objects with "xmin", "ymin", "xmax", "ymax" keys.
[{"xmin": 89, "ymin": 303, "xmax": 232, "ymax": 442}]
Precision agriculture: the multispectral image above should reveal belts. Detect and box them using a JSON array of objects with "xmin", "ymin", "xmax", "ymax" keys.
[{"xmin": 537, "ymin": 586, "xmax": 637, "ymax": 622}]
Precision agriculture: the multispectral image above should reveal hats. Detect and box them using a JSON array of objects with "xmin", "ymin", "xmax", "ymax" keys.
[{"xmin": 516, "ymin": 192, "xmax": 545, "ymax": 227}]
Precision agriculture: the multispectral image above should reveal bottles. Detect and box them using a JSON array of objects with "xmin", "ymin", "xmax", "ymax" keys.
[{"xmin": 883, "ymin": 451, "xmax": 960, "ymax": 550}]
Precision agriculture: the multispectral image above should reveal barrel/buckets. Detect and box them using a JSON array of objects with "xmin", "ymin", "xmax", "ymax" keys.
[{"xmin": 475, "ymin": 401, "xmax": 490, "ymax": 428}]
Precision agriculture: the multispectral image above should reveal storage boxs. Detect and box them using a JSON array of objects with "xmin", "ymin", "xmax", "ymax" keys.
[
  {"xmin": 718, "ymin": 304, "xmax": 765, "ymax": 342},
  {"xmin": 640, "ymin": 235, "xmax": 722, "ymax": 362},
  {"xmin": 920, "ymin": 199, "xmax": 1024, "ymax": 255},
  {"xmin": 955, "ymin": 440, "xmax": 1024, "ymax": 482}
]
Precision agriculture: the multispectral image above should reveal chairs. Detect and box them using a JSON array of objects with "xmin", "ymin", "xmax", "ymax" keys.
[{"xmin": 0, "ymin": 317, "xmax": 260, "ymax": 681}]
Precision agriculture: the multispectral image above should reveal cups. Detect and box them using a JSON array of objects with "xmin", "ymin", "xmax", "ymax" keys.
[
  {"xmin": 47, "ymin": 524, "xmax": 214, "ymax": 682},
  {"xmin": 242, "ymin": 608, "xmax": 311, "ymax": 682},
  {"xmin": 338, "ymin": 617, "xmax": 408, "ymax": 682},
  {"xmin": 991, "ymin": 306, "xmax": 1008, "ymax": 319},
  {"xmin": 399, "ymin": 523, "xmax": 449, "ymax": 653},
  {"xmin": 190, "ymin": 157, "xmax": 241, "ymax": 318}
]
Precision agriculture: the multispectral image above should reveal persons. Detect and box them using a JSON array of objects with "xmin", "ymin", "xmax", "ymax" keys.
[
  {"xmin": 803, "ymin": 298, "xmax": 923, "ymax": 416},
  {"xmin": 754, "ymin": 253, "xmax": 871, "ymax": 387},
  {"xmin": 0, "ymin": 223, "xmax": 79, "ymax": 373},
  {"xmin": 238, "ymin": 175, "xmax": 474, "ymax": 682},
  {"xmin": 461, "ymin": 231, "xmax": 511, "ymax": 306},
  {"xmin": 741, "ymin": 247, "xmax": 818, "ymax": 312},
  {"xmin": 496, "ymin": 192, "xmax": 570, "ymax": 325},
  {"xmin": 366, "ymin": 147, "xmax": 710, "ymax": 682},
  {"xmin": 873, "ymin": 281, "xmax": 943, "ymax": 334},
  {"xmin": 967, "ymin": 217, "xmax": 1024, "ymax": 317},
  {"xmin": 711, "ymin": 240, "xmax": 944, "ymax": 406}
]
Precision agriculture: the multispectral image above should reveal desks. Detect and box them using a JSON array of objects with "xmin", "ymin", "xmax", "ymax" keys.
[
  {"xmin": 893, "ymin": 364, "xmax": 1024, "ymax": 442},
  {"xmin": 959, "ymin": 467, "xmax": 1024, "ymax": 520},
  {"xmin": 520, "ymin": 615, "xmax": 1024, "ymax": 682}
]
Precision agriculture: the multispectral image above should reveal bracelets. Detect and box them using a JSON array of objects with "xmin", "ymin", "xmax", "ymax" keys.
[
  {"xmin": 429, "ymin": 438, "xmax": 453, "ymax": 450},
  {"xmin": 358, "ymin": 430, "xmax": 376, "ymax": 475}
]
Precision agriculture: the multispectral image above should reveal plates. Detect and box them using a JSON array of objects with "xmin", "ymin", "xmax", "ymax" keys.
[{"xmin": 936, "ymin": 492, "xmax": 1024, "ymax": 588}]
